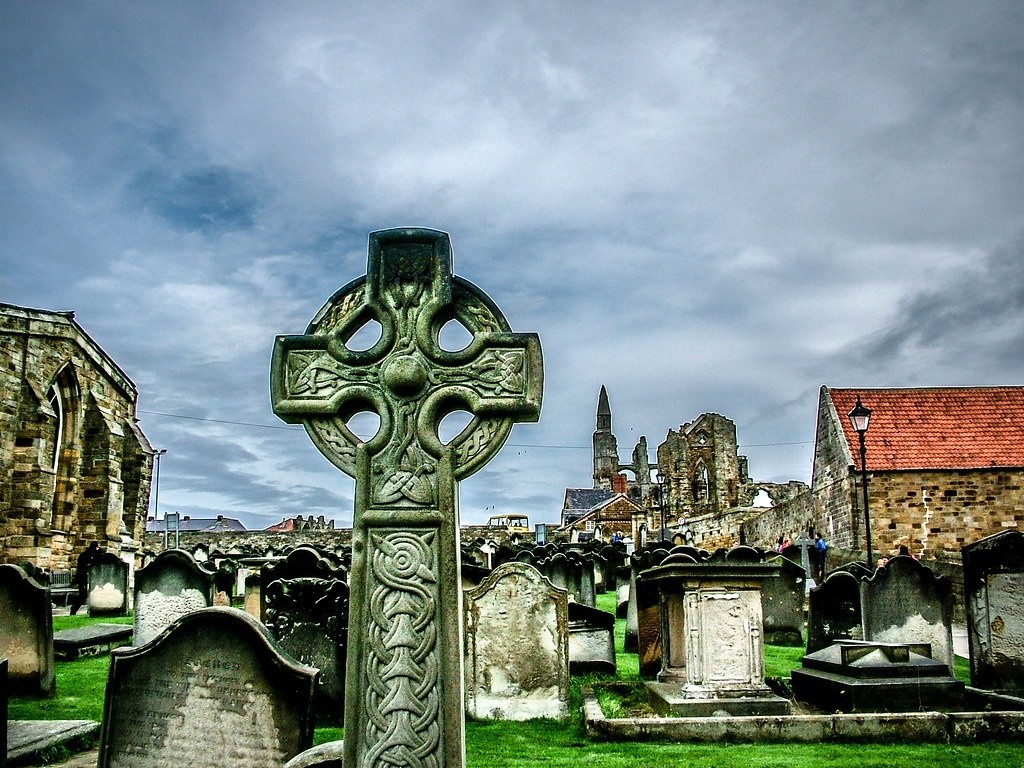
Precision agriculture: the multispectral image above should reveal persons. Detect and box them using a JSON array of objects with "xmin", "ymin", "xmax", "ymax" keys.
[
  {"xmin": 732, "ymin": 541, "xmax": 740, "ymax": 547},
  {"xmin": 776, "ymin": 532, "xmax": 827, "ymax": 583},
  {"xmin": 877, "ymin": 556, "xmax": 889, "ymax": 567}
]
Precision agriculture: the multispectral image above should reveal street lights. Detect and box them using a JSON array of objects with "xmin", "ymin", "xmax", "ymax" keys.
[
  {"xmin": 655, "ymin": 472, "xmax": 667, "ymax": 543},
  {"xmin": 152, "ymin": 449, "xmax": 167, "ymax": 533},
  {"xmin": 846, "ymin": 394, "xmax": 873, "ymax": 569}
]
